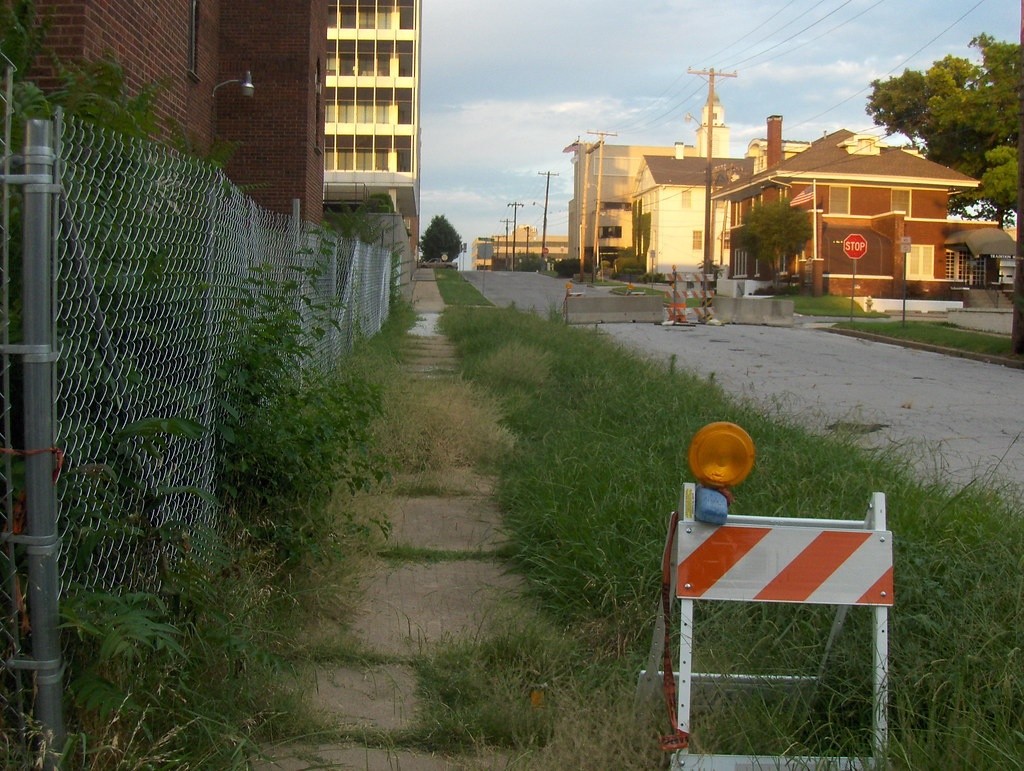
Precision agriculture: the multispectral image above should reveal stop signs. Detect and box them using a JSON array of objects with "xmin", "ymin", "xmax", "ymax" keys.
[{"xmin": 843, "ymin": 233, "xmax": 868, "ymax": 259}]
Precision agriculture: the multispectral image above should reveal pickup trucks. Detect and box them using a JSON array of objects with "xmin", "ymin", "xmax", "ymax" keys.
[{"xmin": 421, "ymin": 258, "xmax": 458, "ymax": 270}]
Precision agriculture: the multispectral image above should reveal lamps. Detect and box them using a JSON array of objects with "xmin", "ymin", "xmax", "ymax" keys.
[{"xmin": 212, "ymin": 71, "xmax": 254, "ymax": 99}]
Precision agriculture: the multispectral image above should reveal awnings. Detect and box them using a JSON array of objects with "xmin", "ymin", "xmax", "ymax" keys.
[{"xmin": 945, "ymin": 227, "xmax": 1016, "ymax": 255}]
